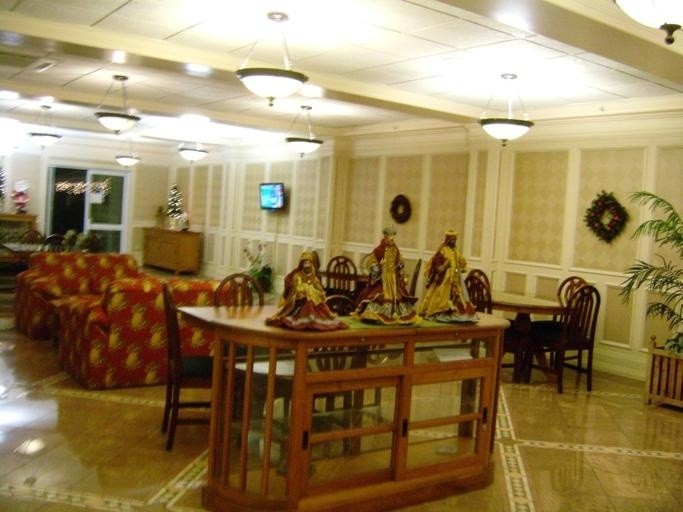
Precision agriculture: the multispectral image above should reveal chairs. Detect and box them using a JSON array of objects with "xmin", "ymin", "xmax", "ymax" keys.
[
  {"xmin": 160, "ymin": 281, "xmax": 214, "ymax": 450},
  {"xmin": 0, "ymin": 212, "xmax": 218, "ymax": 387},
  {"xmin": 462, "ymin": 268, "xmax": 603, "ymax": 396}
]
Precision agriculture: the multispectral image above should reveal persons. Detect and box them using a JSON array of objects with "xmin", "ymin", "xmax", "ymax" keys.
[
  {"xmin": 354, "ymin": 222, "xmax": 423, "ymax": 327},
  {"xmin": 259, "ymin": 250, "xmax": 349, "ymax": 332},
  {"xmin": 416, "ymin": 227, "xmax": 482, "ymax": 326}
]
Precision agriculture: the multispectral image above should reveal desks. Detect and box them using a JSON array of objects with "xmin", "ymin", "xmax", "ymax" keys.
[{"xmin": 177, "ymin": 297, "xmax": 510, "ymax": 512}]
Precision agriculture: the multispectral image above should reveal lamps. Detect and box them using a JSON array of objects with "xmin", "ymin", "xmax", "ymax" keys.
[
  {"xmin": 284, "ymin": 105, "xmax": 322, "ymax": 162},
  {"xmin": 94, "ymin": 74, "xmax": 214, "ymax": 169},
  {"xmin": 480, "ymin": 74, "xmax": 534, "ymax": 146},
  {"xmin": 614, "ymin": 1, "xmax": 682, "ymax": 44},
  {"xmin": 27, "ymin": 102, "xmax": 63, "ymax": 150},
  {"xmin": 235, "ymin": 12, "xmax": 309, "ymax": 104}
]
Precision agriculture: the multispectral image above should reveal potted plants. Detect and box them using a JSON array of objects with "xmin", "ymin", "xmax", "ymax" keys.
[{"xmin": 619, "ymin": 187, "xmax": 682, "ymax": 412}]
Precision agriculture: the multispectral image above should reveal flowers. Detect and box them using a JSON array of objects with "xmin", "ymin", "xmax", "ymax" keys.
[
  {"xmin": 584, "ymin": 189, "xmax": 630, "ymax": 244},
  {"xmin": 388, "ymin": 193, "xmax": 411, "ymax": 222},
  {"xmin": 164, "ymin": 183, "xmax": 185, "ymax": 218}
]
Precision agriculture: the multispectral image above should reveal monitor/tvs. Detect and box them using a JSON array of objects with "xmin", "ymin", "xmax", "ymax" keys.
[{"xmin": 259, "ymin": 183, "xmax": 285, "ymax": 210}]
